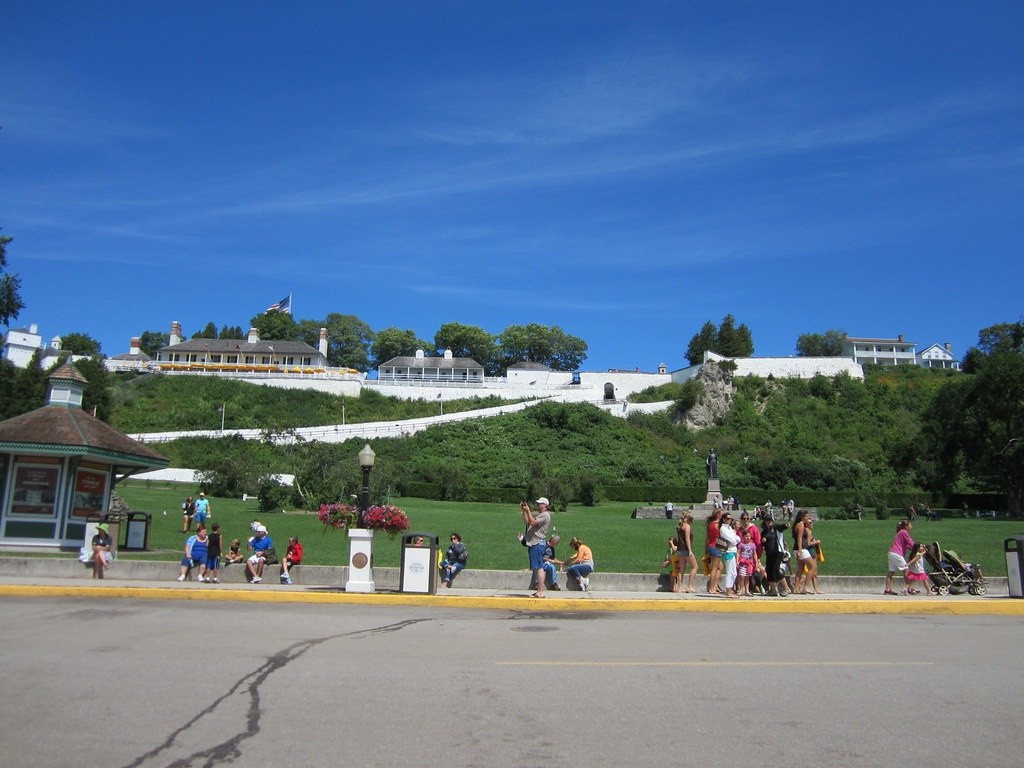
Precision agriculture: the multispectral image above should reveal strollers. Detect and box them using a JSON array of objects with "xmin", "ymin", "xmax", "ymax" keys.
[{"xmin": 922, "ymin": 543, "xmax": 987, "ymax": 596}]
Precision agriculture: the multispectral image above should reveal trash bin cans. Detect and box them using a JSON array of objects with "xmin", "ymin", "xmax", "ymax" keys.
[
  {"xmin": 125, "ymin": 512, "xmax": 151, "ymax": 551},
  {"xmin": 1003, "ymin": 536, "xmax": 1024, "ymax": 597},
  {"xmin": 398, "ymin": 532, "xmax": 440, "ymax": 595},
  {"xmin": 84, "ymin": 512, "xmax": 120, "ymax": 555}
]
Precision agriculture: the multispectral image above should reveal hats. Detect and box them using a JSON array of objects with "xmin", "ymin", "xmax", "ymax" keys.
[
  {"xmin": 96, "ymin": 523, "xmax": 110, "ymax": 534},
  {"xmin": 200, "ymin": 493, "xmax": 205, "ymax": 496},
  {"xmin": 255, "ymin": 525, "xmax": 268, "ymax": 534},
  {"xmin": 535, "ymin": 497, "xmax": 550, "ymax": 505}
]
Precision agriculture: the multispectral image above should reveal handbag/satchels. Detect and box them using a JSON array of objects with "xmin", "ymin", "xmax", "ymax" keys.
[
  {"xmin": 456, "ymin": 541, "xmax": 470, "ymax": 561},
  {"xmin": 701, "ymin": 555, "xmax": 712, "ymax": 575},
  {"xmin": 521, "ymin": 536, "xmax": 529, "ymax": 547},
  {"xmin": 815, "ymin": 544, "xmax": 824, "ymax": 562},
  {"xmin": 671, "ymin": 556, "xmax": 681, "ymax": 576},
  {"xmin": 715, "ymin": 525, "xmax": 728, "ymax": 551},
  {"xmin": 781, "ymin": 551, "xmax": 789, "ymax": 563}
]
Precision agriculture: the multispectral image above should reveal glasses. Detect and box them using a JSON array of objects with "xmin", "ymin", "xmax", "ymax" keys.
[
  {"xmin": 725, "ymin": 517, "xmax": 813, "ymax": 524},
  {"xmin": 419, "ymin": 538, "xmax": 456, "ymax": 542}
]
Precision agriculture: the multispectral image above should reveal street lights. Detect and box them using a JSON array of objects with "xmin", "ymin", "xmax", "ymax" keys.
[{"xmin": 355, "ymin": 444, "xmax": 375, "ymax": 530}]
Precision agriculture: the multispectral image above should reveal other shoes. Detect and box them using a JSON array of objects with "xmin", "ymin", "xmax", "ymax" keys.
[
  {"xmin": 579, "ymin": 578, "xmax": 589, "ymax": 592},
  {"xmin": 95, "ymin": 573, "xmax": 100, "ymax": 579},
  {"xmin": 529, "ymin": 591, "xmax": 545, "ymax": 598},
  {"xmin": 884, "ymin": 588, "xmax": 921, "ymax": 595},
  {"xmin": 441, "ymin": 587, "xmax": 448, "ymax": 592},
  {"xmin": 451, "ymin": 567, "xmax": 456, "ymax": 574},
  {"xmin": 183, "ymin": 529, "xmax": 189, "ymax": 533},
  {"xmin": 536, "ymin": 583, "xmax": 561, "ymax": 591},
  {"xmin": 901, "ymin": 589, "xmax": 937, "ymax": 596},
  {"xmin": 672, "ymin": 585, "xmax": 824, "ymax": 599},
  {"xmin": 250, "ymin": 576, "xmax": 262, "ymax": 584},
  {"xmin": 205, "ymin": 577, "xmax": 217, "ymax": 582},
  {"xmin": 105, "ymin": 566, "xmax": 110, "ymax": 570},
  {"xmin": 229, "ymin": 559, "xmax": 234, "ymax": 563},
  {"xmin": 178, "ymin": 575, "xmax": 205, "ymax": 582},
  {"xmin": 281, "ymin": 573, "xmax": 292, "ymax": 584}
]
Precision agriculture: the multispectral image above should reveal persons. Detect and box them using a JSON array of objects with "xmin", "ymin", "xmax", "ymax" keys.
[
  {"xmin": 660, "ymin": 493, "xmax": 825, "ymax": 598},
  {"xmin": 204, "ymin": 522, "xmax": 224, "ymax": 583},
  {"xmin": 174, "ymin": 525, "xmax": 209, "ymax": 582},
  {"xmin": 904, "ymin": 542, "xmax": 938, "ymax": 596},
  {"xmin": 542, "ymin": 534, "xmax": 572, "ymax": 591},
  {"xmin": 520, "ymin": 497, "xmax": 551, "ymax": 598},
  {"xmin": 224, "ymin": 537, "xmax": 244, "ymax": 563},
  {"xmin": 438, "ymin": 532, "xmax": 469, "ymax": 589},
  {"xmin": 706, "ymin": 449, "xmax": 718, "ymax": 478},
  {"xmin": 91, "ymin": 523, "xmax": 113, "ymax": 579},
  {"xmin": 279, "ymin": 536, "xmax": 303, "ymax": 583},
  {"xmin": 413, "ymin": 536, "xmax": 424, "ymax": 546},
  {"xmin": 958, "ymin": 555, "xmax": 989, "ymax": 586},
  {"xmin": 908, "ymin": 504, "xmax": 943, "ymax": 522},
  {"xmin": 565, "ymin": 537, "xmax": 594, "ymax": 591},
  {"xmin": 246, "ymin": 520, "xmax": 274, "ymax": 583},
  {"xmin": 181, "ymin": 492, "xmax": 211, "ymax": 532},
  {"xmin": 883, "ymin": 520, "xmax": 926, "ymax": 596},
  {"xmin": 854, "ymin": 502, "xmax": 863, "ymax": 521}
]
